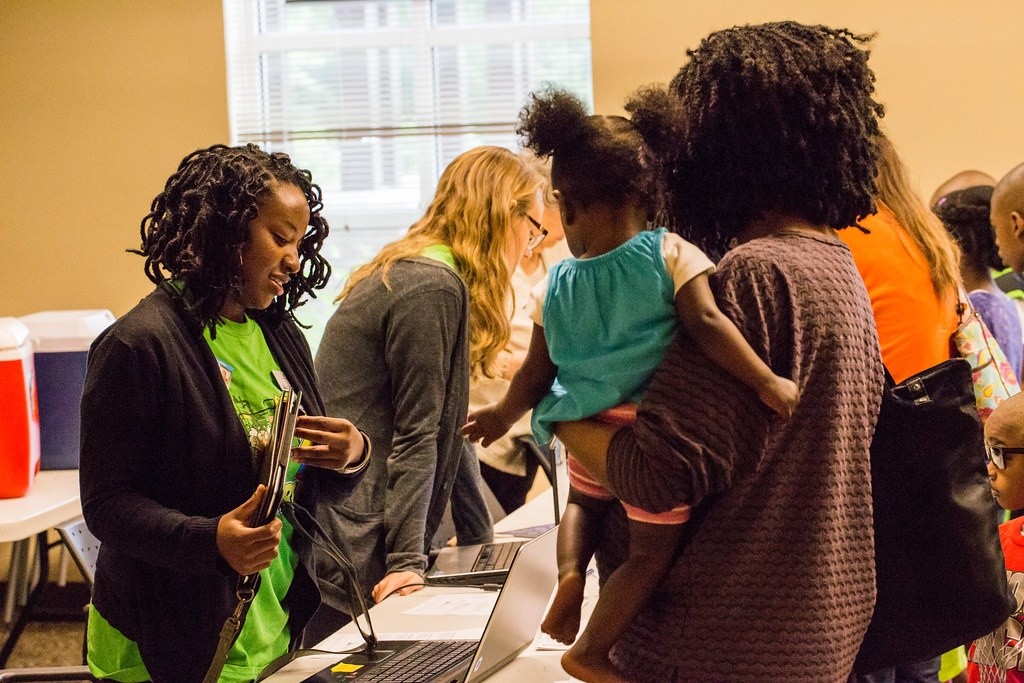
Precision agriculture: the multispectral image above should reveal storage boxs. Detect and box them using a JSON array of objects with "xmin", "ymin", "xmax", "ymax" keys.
[
  {"xmin": 22, "ymin": 308, "xmax": 119, "ymax": 472},
  {"xmin": 0, "ymin": 316, "xmax": 44, "ymax": 501}
]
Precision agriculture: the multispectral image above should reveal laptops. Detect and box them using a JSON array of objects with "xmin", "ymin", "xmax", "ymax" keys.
[
  {"xmin": 424, "ymin": 435, "xmax": 570, "ymax": 586},
  {"xmin": 299, "ymin": 522, "xmax": 560, "ymax": 682}
]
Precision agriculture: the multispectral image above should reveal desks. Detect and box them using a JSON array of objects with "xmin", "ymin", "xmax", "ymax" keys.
[
  {"xmin": 254, "ymin": 475, "xmax": 603, "ymax": 683},
  {"xmin": 0, "ymin": 464, "xmax": 88, "ymax": 670}
]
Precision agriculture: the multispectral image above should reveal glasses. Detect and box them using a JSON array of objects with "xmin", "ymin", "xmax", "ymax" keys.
[
  {"xmin": 984, "ymin": 439, "xmax": 1024, "ymax": 471},
  {"xmin": 523, "ymin": 212, "xmax": 547, "ymax": 250}
]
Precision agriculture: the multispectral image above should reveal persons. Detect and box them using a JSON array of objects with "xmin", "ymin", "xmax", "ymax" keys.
[
  {"xmin": 66, "ymin": 137, "xmax": 381, "ymax": 683},
  {"xmin": 300, "ymin": 137, "xmax": 547, "ymax": 650},
  {"xmin": 828, "ymin": 126, "xmax": 969, "ymax": 682},
  {"xmin": 544, "ymin": 13, "xmax": 887, "ymax": 683},
  {"xmin": 987, "ymin": 156, "xmax": 1024, "ymax": 275},
  {"xmin": 965, "ymin": 395, "xmax": 1024, "ymax": 681},
  {"xmin": 931, "ymin": 180, "xmax": 1024, "ymax": 391},
  {"xmin": 458, "ymin": 78, "xmax": 804, "ymax": 681},
  {"xmin": 464, "ymin": 150, "xmax": 585, "ymax": 513},
  {"xmin": 926, "ymin": 159, "xmax": 1023, "ymax": 343}
]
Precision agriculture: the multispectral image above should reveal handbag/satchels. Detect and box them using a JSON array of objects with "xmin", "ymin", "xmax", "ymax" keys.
[
  {"xmin": 852, "ymin": 358, "xmax": 1019, "ymax": 674},
  {"xmin": 955, "ymin": 286, "xmax": 1022, "ymax": 422}
]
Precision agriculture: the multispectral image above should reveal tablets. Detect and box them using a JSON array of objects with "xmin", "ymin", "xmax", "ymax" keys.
[{"xmin": 236, "ymin": 387, "xmax": 303, "ymax": 593}]
